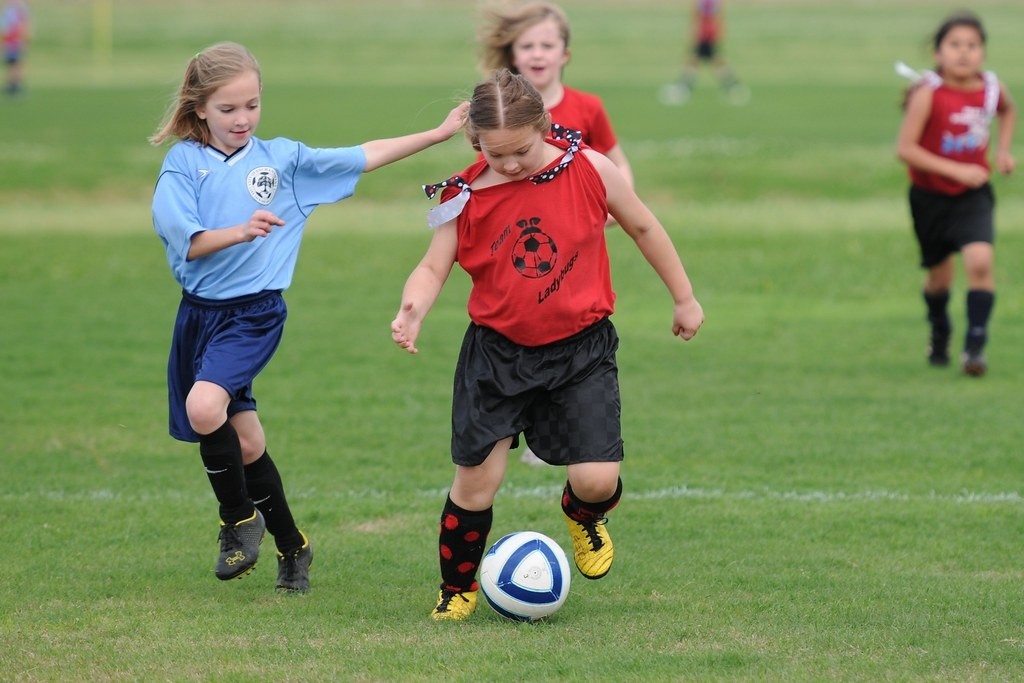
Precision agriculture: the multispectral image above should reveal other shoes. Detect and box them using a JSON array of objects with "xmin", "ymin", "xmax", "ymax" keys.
[
  {"xmin": 965, "ymin": 358, "xmax": 986, "ymax": 376},
  {"xmin": 931, "ymin": 346, "xmax": 949, "ymax": 366}
]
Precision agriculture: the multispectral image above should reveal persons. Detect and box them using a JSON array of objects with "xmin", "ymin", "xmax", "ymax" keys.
[
  {"xmin": 663, "ymin": 0, "xmax": 738, "ymax": 97},
  {"xmin": 0, "ymin": 0, "xmax": 29, "ymax": 92},
  {"xmin": 477, "ymin": 0, "xmax": 632, "ymax": 462},
  {"xmin": 150, "ymin": 39, "xmax": 473, "ymax": 593},
  {"xmin": 897, "ymin": 11, "xmax": 1016, "ymax": 376},
  {"xmin": 392, "ymin": 67, "xmax": 704, "ymax": 621}
]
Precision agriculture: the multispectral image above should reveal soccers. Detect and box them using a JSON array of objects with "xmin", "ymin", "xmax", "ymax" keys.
[{"xmin": 480, "ymin": 531, "xmax": 572, "ymax": 622}]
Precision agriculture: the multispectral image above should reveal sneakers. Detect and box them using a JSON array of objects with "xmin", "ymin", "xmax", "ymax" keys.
[
  {"xmin": 215, "ymin": 507, "xmax": 265, "ymax": 581},
  {"xmin": 431, "ymin": 590, "xmax": 477, "ymax": 621},
  {"xmin": 275, "ymin": 531, "xmax": 314, "ymax": 592},
  {"xmin": 561, "ymin": 510, "xmax": 614, "ymax": 580}
]
